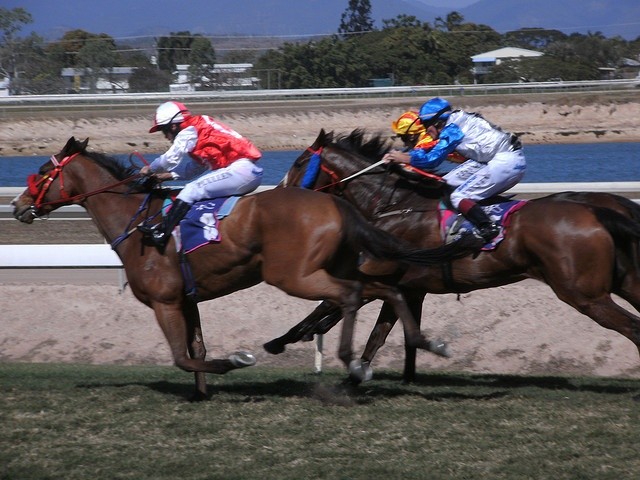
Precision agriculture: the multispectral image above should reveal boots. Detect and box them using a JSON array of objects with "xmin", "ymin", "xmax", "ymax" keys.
[
  {"xmin": 137, "ymin": 199, "xmax": 191, "ymax": 248},
  {"xmin": 458, "ymin": 204, "xmax": 500, "ymax": 248}
]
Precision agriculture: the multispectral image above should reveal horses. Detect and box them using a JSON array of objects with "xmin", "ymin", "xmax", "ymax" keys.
[
  {"xmin": 263, "ymin": 127, "xmax": 640, "ymax": 395},
  {"xmin": 10, "ymin": 136, "xmax": 484, "ymax": 400},
  {"xmin": 336, "ymin": 127, "xmax": 640, "ymax": 382}
]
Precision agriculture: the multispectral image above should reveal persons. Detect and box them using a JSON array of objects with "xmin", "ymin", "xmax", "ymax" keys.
[
  {"xmin": 392, "ymin": 110, "xmax": 468, "ymax": 178},
  {"xmin": 135, "ymin": 101, "xmax": 265, "ymax": 253},
  {"xmin": 383, "ymin": 96, "xmax": 529, "ymax": 251}
]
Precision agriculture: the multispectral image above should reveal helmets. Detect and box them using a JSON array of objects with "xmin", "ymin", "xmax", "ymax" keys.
[
  {"xmin": 391, "ymin": 111, "xmax": 426, "ymax": 135},
  {"xmin": 417, "ymin": 97, "xmax": 452, "ymax": 124},
  {"xmin": 149, "ymin": 101, "xmax": 192, "ymax": 133}
]
